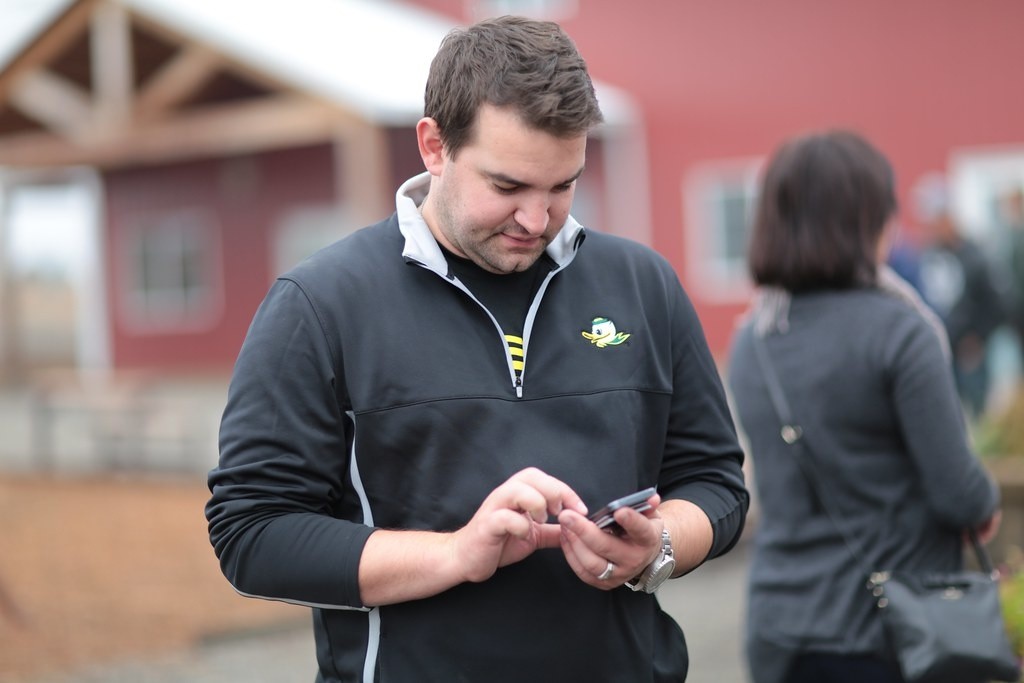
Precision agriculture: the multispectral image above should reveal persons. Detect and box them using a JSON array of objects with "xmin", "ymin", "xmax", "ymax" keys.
[
  {"xmin": 721, "ymin": 128, "xmax": 1001, "ymax": 683},
  {"xmin": 204, "ymin": 15, "xmax": 750, "ymax": 683}
]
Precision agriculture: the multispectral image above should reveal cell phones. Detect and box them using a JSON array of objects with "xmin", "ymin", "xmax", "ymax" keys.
[{"xmin": 589, "ymin": 487, "xmax": 657, "ymax": 523}]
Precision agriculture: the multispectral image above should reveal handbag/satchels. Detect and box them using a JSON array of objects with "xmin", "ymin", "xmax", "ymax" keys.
[{"xmin": 869, "ymin": 569, "xmax": 1022, "ymax": 683}]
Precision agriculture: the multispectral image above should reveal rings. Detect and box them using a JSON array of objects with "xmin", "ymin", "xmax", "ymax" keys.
[{"xmin": 597, "ymin": 563, "xmax": 614, "ymax": 581}]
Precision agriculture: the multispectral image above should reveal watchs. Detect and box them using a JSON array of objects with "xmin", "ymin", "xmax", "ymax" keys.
[{"xmin": 624, "ymin": 532, "xmax": 675, "ymax": 594}]
